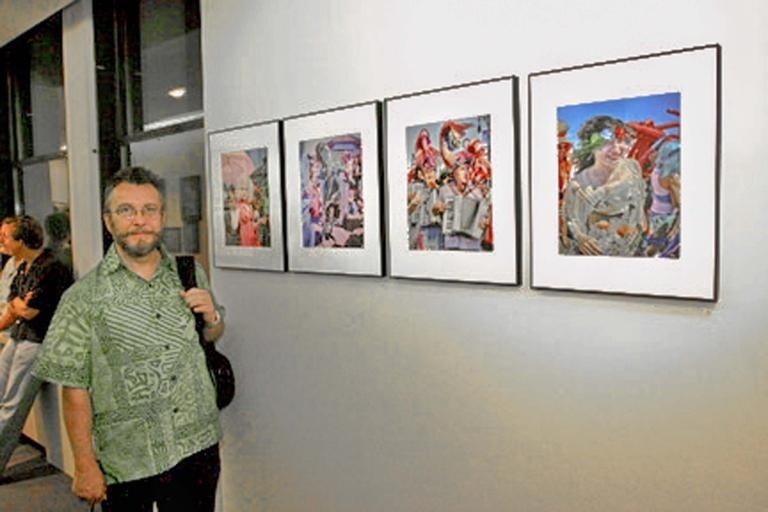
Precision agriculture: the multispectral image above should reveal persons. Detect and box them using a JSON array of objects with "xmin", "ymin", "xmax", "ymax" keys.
[
  {"xmin": 0, "ymin": 215, "xmax": 76, "ymax": 478},
  {"xmin": 44, "ymin": 214, "xmax": 73, "ymax": 272},
  {"xmin": 562, "ymin": 113, "xmax": 648, "ymax": 258},
  {"xmin": 646, "ymin": 140, "xmax": 681, "ymax": 259},
  {"xmin": 30, "ymin": 165, "xmax": 228, "ymax": 511},
  {"xmin": 407, "ymin": 155, "xmax": 493, "ymax": 249},
  {"xmin": 0, "ymin": 216, "xmax": 25, "ymax": 353}
]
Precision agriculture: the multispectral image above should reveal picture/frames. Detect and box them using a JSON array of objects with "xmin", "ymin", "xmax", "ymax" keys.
[
  {"xmin": 281, "ymin": 97, "xmax": 385, "ymax": 282},
  {"xmin": 380, "ymin": 73, "xmax": 524, "ymax": 289},
  {"xmin": 206, "ymin": 117, "xmax": 287, "ymax": 275},
  {"xmin": 525, "ymin": 41, "xmax": 722, "ymax": 301}
]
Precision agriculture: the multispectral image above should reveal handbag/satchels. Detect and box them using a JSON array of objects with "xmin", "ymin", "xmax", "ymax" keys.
[{"xmin": 204, "ymin": 341, "xmax": 235, "ymax": 409}]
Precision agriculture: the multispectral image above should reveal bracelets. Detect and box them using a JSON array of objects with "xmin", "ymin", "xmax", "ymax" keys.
[{"xmin": 206, "ymin": 304, "xmax": 226, "ymax": 328}]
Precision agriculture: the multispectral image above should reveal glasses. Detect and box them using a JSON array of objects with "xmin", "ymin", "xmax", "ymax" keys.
[{"xmin": 109, "ymin": 205, "xmax": 161, "ymax": 218}]
